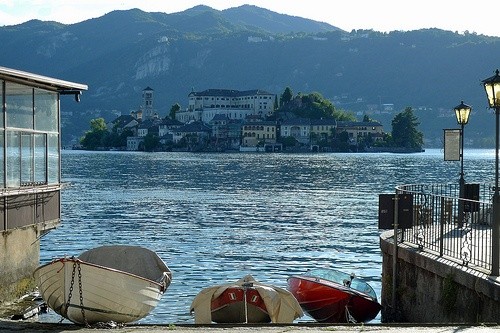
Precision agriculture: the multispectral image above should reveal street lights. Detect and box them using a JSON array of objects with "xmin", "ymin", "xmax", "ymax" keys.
[
  {"xmin": 455, "ymin": 99, "xmax": 471, "ymax": 229},
  {"xmin": 480, "ymin": 69, "xmax": 500, "ymax": 276}
]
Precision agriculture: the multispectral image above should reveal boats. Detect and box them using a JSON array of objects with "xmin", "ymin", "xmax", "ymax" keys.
[
  {"xmin": 285, "ymin": 267, "xmax": 383, "ymax": 323},
  {"xmin": 188, "ymin": 272, "xmax": 282, "ymax": 324},
  {"xmin": 31, "ymin": 243, "xmax": 174, "ymax": 324}
]
